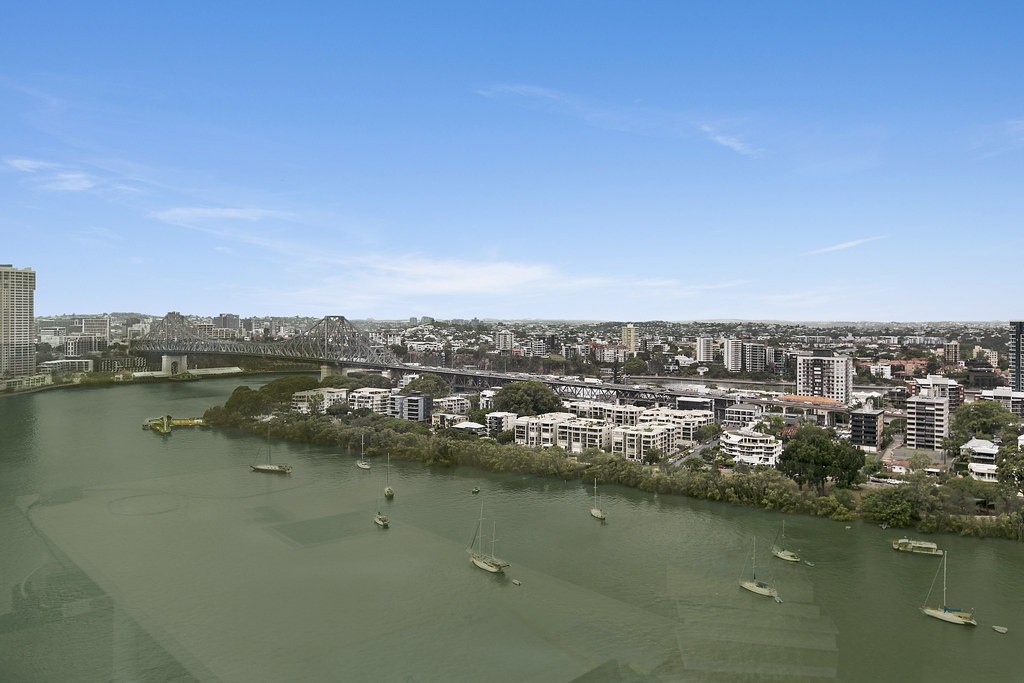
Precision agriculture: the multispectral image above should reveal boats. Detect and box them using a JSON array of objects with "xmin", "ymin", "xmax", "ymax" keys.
[
  {"xmin": 245, "ymin": 421, "xmax": 610, "ymax": 585},
  {"xmin": 732, "ymin": 512, "xmax": 1009, "ymax": 635}
]
